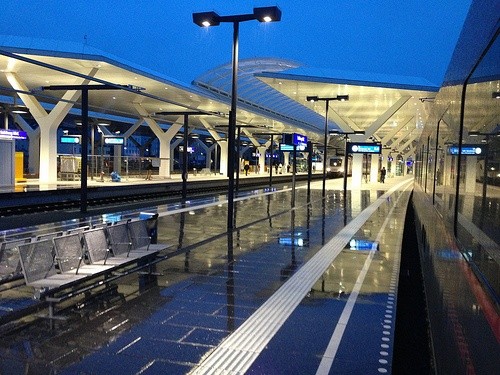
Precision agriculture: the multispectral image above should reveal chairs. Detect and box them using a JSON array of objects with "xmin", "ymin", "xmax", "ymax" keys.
[{"xmin": 0, "ymin": 218, "xmax": 174, "ymax": 330}]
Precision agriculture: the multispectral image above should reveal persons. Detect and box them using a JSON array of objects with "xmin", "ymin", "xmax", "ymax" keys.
[
  {"xmin": 244, "ymin": 163, "xmax": 249, "ymax": 176},
  {"xmin": 287, "ymin": 164, "xmax": 290, "ymax": 172},
  {"xmin": 437, "ymin": 169, "xmax": 441, "ymax": 186},
  {"xmin": 380, "ymin": 167, "xmax": 386, "ymax": 183},
  {"xmin": 275, "ymin": 163, "xmax": 282, "ymax": 174}
]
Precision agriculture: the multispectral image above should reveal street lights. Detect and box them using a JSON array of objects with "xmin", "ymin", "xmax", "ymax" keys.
[
  {"xmin": 328, "ymin": 131, "xmax": 366, "ymax": 189},
  {"xmin": 193, "ymin": 5, "xmax": 282, "ymax": 231},
  {"xmin": 306, "ymin": 95, "xmax": 350, "ymax": 197},
  {"xmin": 468, "ymin": 131, "xmax": 500, "ymax": 232}
]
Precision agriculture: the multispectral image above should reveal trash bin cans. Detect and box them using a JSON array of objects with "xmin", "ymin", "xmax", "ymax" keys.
[
  {"xmin": 102, "ymin": 211, "xmax": 159, "ymax": 258},
  {"xmin": 193, "ymin": 168, "xmax": 197, "ymax": 176}
]
